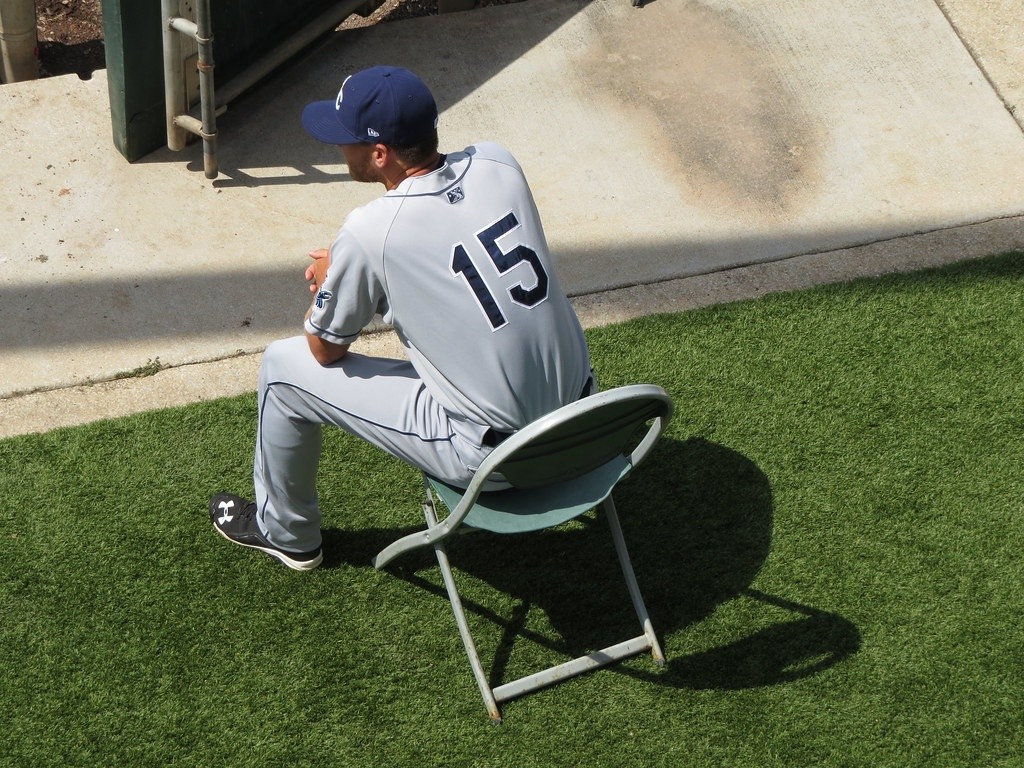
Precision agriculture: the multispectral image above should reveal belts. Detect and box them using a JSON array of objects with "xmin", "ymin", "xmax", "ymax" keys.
[{"xmin": 480, "ymin": 372, "xmax": 594, "ymax": 448}]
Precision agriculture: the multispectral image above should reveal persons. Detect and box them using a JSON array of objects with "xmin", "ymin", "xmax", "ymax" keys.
[{"xmin": 208, "ymin": 65, "xmax": 599, "ymax": 571}]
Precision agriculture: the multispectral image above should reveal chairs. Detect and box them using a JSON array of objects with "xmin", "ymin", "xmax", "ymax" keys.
[{"xmin": 372, "ymin": 385, "xmax": 675, "ymax": 723}]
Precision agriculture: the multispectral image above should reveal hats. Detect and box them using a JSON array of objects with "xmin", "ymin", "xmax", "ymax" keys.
[{"xmin": 301, "ymin": 66, "xmax": 439, "ymax": 144}]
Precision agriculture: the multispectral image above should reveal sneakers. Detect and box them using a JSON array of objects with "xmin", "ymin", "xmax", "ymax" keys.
[{"xmin": 209, "ymin": 492, "xmax": 323, "ymax": 571}]
